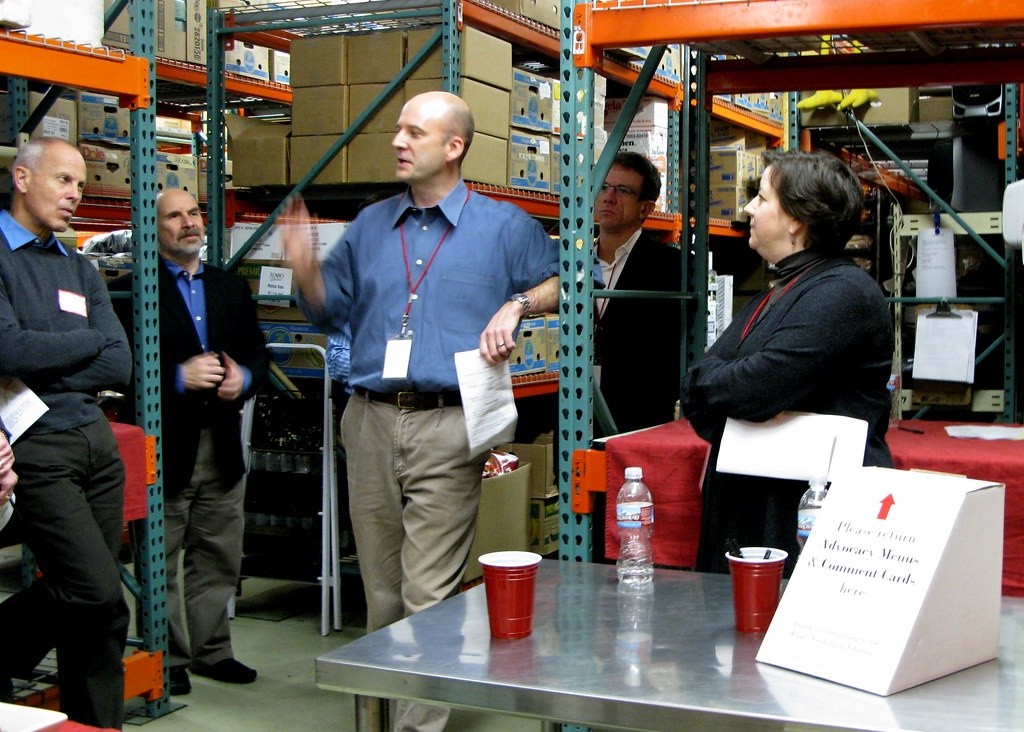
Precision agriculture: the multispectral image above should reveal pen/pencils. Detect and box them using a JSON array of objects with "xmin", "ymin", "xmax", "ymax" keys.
[{"xmin": 726, "ymin": 537, "xmax": 771, "ymax": 559}]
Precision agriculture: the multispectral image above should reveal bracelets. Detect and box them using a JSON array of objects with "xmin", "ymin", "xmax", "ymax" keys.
[{"xmin": 0, "ymin": 428, "xmax": 8, "ymax": 440}]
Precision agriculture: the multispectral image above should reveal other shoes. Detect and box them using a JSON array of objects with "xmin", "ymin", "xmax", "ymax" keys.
[
  {"xmin": 168, "ymin": 665, "xmax": 192, "ymax": 695},
  {"xmin": 190, "ymin": 658, "xmax": 258, "ymax": 684}
]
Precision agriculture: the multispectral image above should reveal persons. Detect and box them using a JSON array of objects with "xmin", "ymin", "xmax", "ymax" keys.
[
  {"xmin": 110, "ymin": 186, "xmax": 272, "ymax": 696},
  {"xmin": 684, "ymin": 148, "xmax": 894, "ymax": 577},
  {"xmin": 279, "ymin": 93, "xmax": 604, "ymax": 732},
  {"xmin": 594, "ymin": 153, "xmax": 694, "ymax": 440},
  {"xmin": 0, "ymin": 135, "xmax": 140, "ymax": 730}
]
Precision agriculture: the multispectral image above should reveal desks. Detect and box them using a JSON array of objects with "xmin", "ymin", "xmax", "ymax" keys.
[
  {"xmin": 591, "ymin": 416, "xmax": 1024, "ymax": 596},
  {"xmin": 315, "ymin": 559, "xmax": 1024, "ymax": 732}
]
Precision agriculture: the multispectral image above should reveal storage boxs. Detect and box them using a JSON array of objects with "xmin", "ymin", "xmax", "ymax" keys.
[
  {"xmin": 222, "ymin": 1, "xmax": 954, "ymax": 377},
  {"xmin": 0, "ymin": 90, "xmax": 199, "ymax": 369},
  {"xmin": 462, "ymin": 429, "xmax": 559, "ymax": 585},
  {"xmin": 101, "ymin": 0, "xmax": 207, "ymax": 65}
]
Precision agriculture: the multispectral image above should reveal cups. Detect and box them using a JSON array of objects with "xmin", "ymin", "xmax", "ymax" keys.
[
  {"xmin": 725, "ymin": 546, "xmax": 788, "ymax": 634},
  {"xmin": 478, "ymin": 550, "xmax": 543, "ymax": 639}
]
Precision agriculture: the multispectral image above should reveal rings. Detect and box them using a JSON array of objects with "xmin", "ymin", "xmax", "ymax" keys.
[
  {"xmin": 497, "ymin": 341, "xmax": 505, "ymax": 348},
  {"xmin": 5, "ymin": 493, "xmax": 11, "ymax": 501}
]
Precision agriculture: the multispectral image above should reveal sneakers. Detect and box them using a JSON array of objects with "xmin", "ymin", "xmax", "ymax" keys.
[{"xmin": 396, "ymin": 699, "xmax": 450, "ymax": 732}]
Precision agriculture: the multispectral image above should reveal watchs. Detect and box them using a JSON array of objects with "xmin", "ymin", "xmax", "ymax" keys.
[{"xmin": 507, "ymin": 293, "xmax": 530, "ymax": 315}]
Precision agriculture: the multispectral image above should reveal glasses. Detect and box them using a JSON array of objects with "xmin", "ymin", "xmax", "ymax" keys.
[{"xmin": 601, "ymin": 183, "xmax": 647, "ymax": 200}]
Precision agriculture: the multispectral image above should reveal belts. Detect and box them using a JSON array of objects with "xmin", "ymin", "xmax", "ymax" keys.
[{"xmin": 353, "ymin": 384, "xmax": 462, "ymax": 410}]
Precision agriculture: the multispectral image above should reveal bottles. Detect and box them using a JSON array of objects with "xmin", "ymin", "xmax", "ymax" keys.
[
  {"xmin": 614, "ymin": 467, "xmax": 655, "ymax": 584},
  {"xmin": 796, "ymin": 476, "xmax": 829, "ymax": 556}
]
